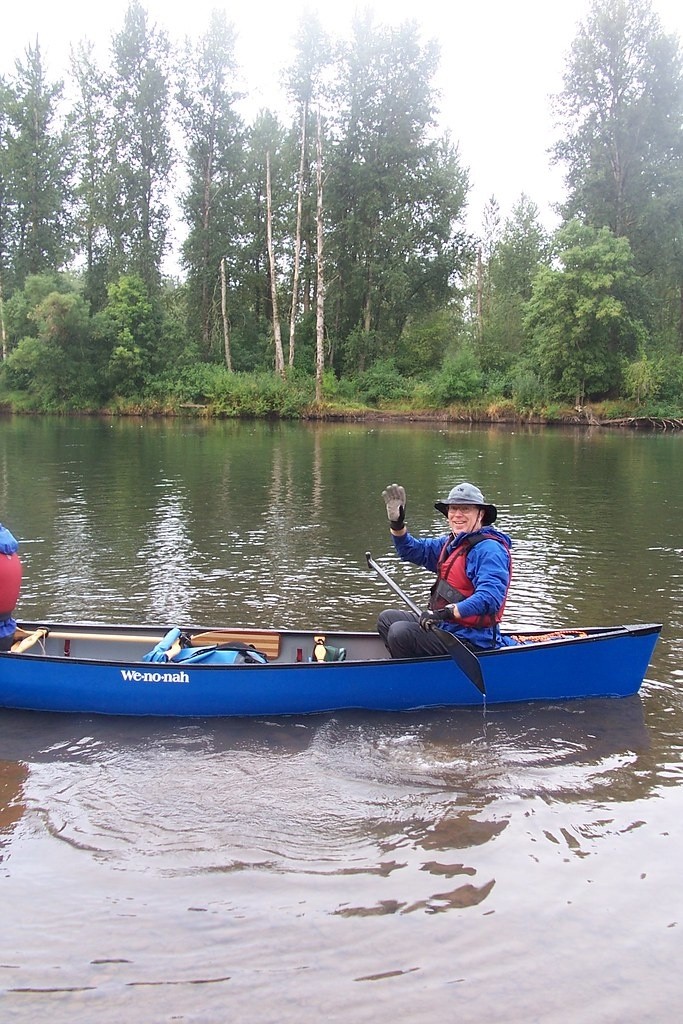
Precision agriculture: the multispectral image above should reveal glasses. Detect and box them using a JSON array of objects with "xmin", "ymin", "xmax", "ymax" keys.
[{"xmin": 448, "ymin": 506, "xmax": 477, "ymax": 514}]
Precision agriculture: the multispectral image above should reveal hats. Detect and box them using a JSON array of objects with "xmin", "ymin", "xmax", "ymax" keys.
[{"xmin": 434, "ymin": 482, "xmax": 497, "ymax": 527}]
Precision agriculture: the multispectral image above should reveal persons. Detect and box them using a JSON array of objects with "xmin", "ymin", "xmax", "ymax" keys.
[
  {"xmin": 0, "ymin": 522, "xmax": 22, "ymax": 651},
  {"xmin": 378, "ymin": 483, "xmax": 512, "ymax": 658}
]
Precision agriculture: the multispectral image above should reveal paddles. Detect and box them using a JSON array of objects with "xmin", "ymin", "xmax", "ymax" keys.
[
  {"xmin": 17, "ymin": 629, "xmax": 280, "ymax": 660},
  {"xmin": 365, "ymin": 552, "xmax": 485, "ymax": 693}
]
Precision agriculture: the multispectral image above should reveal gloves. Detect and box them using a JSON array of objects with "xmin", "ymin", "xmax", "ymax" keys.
[
  {"xmin": 419, "ymin": 605, "xmax": 455, "ymax": 632},
  {"xmin": 381, "ymin": 484, "xmax": 406, "ymax": 530}
]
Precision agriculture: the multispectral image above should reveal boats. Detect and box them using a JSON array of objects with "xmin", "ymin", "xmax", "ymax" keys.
[{"xmin": 0, "ymin": 619, "xmax": 663, "ymax": 718}]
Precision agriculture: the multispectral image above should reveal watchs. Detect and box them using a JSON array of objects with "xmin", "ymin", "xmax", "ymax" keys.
[{"xmin": 444, "ymin": 604, "xmax": 456, "ymax": 618}]
